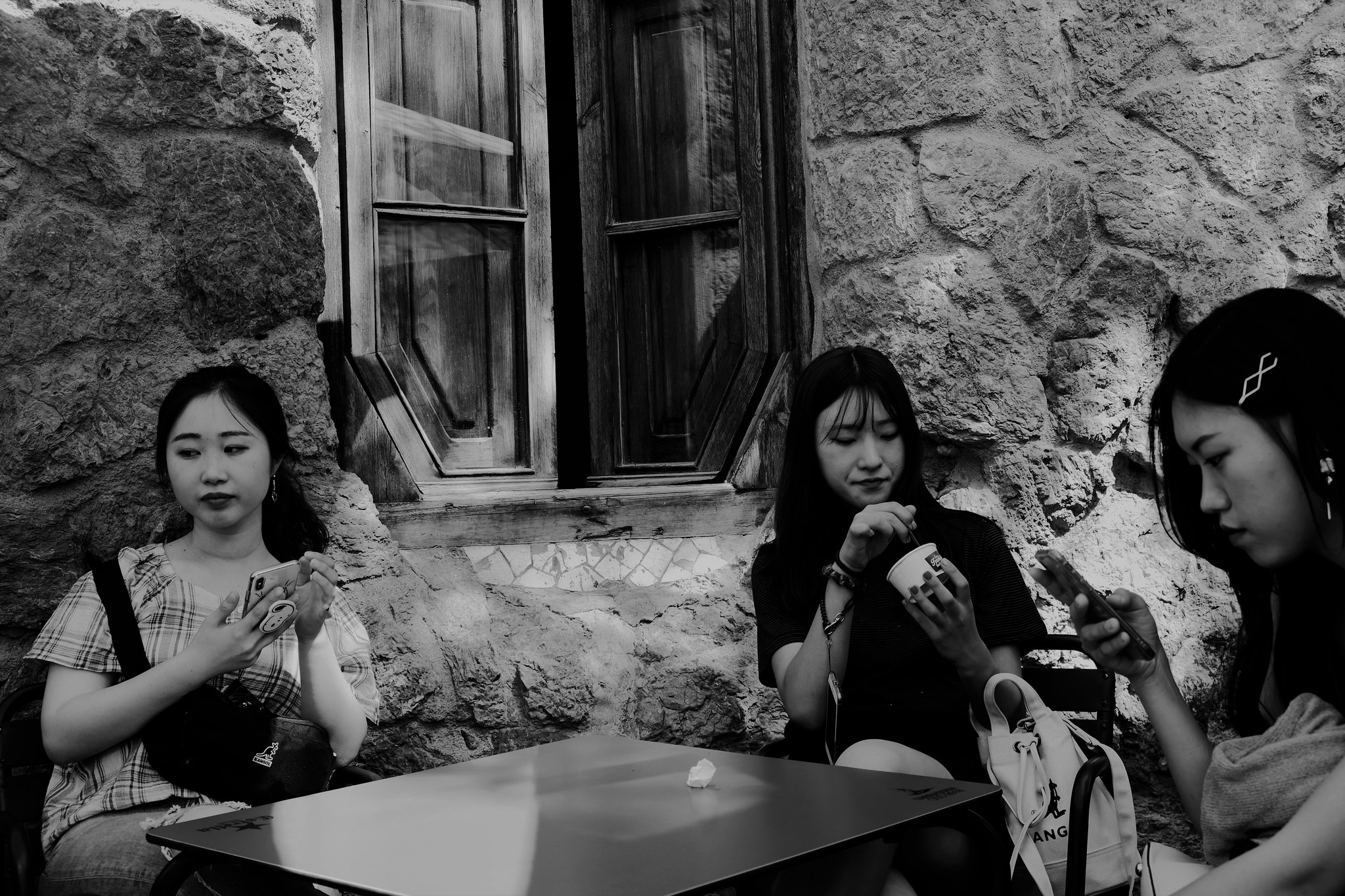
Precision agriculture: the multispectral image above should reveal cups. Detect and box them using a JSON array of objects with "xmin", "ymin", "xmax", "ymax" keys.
[{"xmin": 886, "ymin": 543, "xmax": 950, "ymax": 604}]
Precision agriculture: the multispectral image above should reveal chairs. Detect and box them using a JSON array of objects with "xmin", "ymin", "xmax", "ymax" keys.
[
  {"xmin": 0, "ymin": 682, "xmax": 382, "ymax": 896},
  {"xmin": 758, "ymin": 633, "xmax": 1112, "ymax": 896}
]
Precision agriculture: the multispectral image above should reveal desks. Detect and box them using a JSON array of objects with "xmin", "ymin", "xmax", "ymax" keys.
[{"xmin": 145, "ymin": 732, "xmax": 1004, "ymax": 896}]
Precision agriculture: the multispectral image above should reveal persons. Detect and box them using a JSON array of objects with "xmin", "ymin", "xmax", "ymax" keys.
[
  {"xmin": 22, "ymin": 363, "xmax": 381, "ymax": 896},
  {"xmin": 1025, "ymin": 288, "xmax": 1345, "ymax": 896},
  {"xmin": 751, "ymin": 344, "xmax": 1049, "ymax": 896}
]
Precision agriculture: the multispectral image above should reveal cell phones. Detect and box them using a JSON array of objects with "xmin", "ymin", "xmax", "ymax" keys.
[
  {"xmin": 242, "ymin": 560, "xmax": 302, "ymax": 620},
  {"xmin": 1035, "ymin": 547, "xmax": 1154, "ymax": 661}
]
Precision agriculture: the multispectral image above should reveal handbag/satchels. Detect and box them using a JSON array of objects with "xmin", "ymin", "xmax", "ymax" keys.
[
  {"xmin": 95, "ymin": 557, "xmax": 325, "ymax": 801},
  {"xmin": 973, "ymin": 669, "xmax": 1143, "ymax": 896}
]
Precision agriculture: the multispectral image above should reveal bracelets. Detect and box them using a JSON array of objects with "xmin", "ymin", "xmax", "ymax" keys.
[
  {"xmin": 834, "ymin": 550, "xmax": 859, "ymax": 580},
  {"xmin": 821, "ymin": 565, "xmax": 860, "ymax": 592}
]
